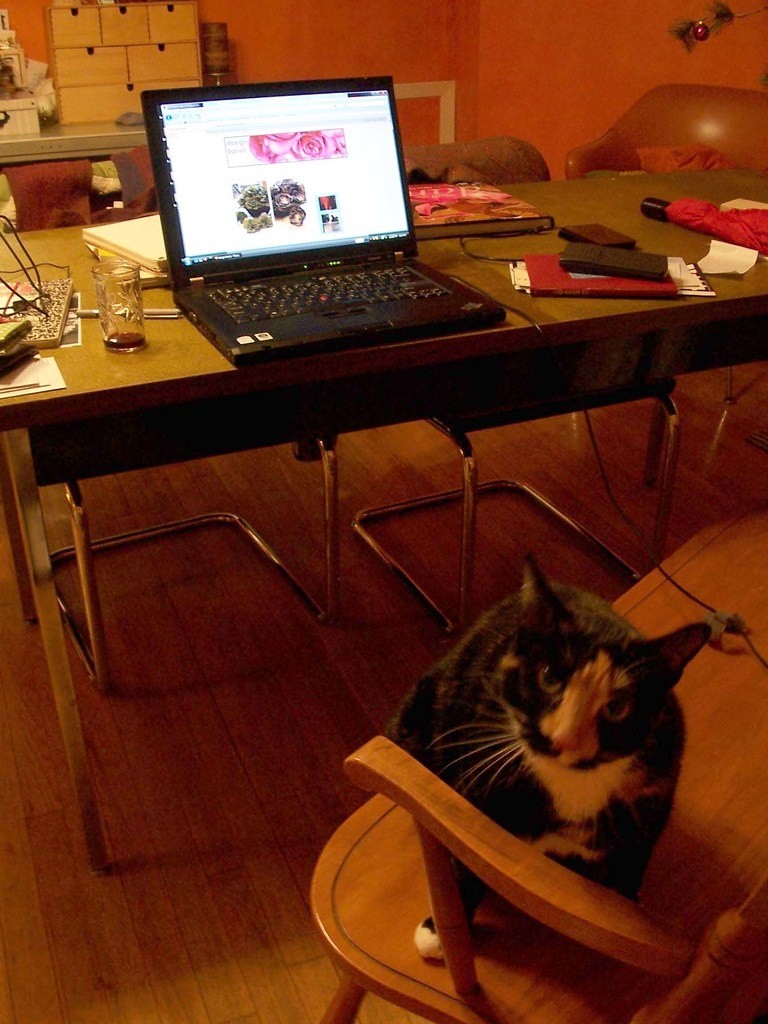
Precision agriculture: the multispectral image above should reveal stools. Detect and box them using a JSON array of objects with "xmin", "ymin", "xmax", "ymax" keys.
[
  {"xmin": 349, "ymin": 383, "xmax": 682, "ymax": 637},
  {"xmin": 49, "ymin": 434, "xmax": 339, "ymax": 690}
]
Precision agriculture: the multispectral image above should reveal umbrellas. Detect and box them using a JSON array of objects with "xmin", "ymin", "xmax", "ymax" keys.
[{"xmin": 640, "ymin": 197, "xmax": 768, "ymax": 256}]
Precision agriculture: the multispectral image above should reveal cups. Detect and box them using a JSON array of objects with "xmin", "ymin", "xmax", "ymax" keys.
[{"xmin": 91, "ymin": 260, "xmax": 147, "ymax": 354}]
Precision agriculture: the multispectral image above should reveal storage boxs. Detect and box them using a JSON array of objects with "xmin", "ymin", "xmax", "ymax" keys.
[{"xmin": 0, "ymin": 97, "xmax": 41, "ymax": 135}]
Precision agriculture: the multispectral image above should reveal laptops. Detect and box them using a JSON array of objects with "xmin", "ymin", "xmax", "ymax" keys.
[{"xmin": 140, "ymin": 75, "xmax": 507, "ymax": 366}]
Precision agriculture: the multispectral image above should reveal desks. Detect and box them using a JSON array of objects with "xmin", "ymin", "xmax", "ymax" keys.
[{"xmin": 0, "ymin": 166, "xmax": 768, "ymax": 877}]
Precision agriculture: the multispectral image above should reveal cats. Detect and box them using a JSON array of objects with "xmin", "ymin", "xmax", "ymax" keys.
[{"xmin": 385, "ymin": 547, "xmax": 713, "ymax": 959}]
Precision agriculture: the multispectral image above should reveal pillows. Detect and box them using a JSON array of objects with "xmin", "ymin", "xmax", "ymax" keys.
[
  {"xmin": 635, "ymin": 144, "xmax": 741, "ymax": 173},
  {"xmin": 1, "ymin": 159, "xmax": 93, "ymax": 233},
  {"xmin": 108, "ymin": 145, "xmax": 156, "ymax": 208}
]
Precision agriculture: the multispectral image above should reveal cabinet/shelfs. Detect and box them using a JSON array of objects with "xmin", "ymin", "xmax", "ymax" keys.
[{"xmin": 47, "ymin": 0, "xmax": 204, "ymax": 126}]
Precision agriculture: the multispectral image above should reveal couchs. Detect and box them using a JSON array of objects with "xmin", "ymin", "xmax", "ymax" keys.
[
  {"xmin": 564, "ymin": 82, "xmax": 768, "ymax": 180},
  {"xmin": 403, "ymin": 136, "xmax": 551, "ymax": 185}
]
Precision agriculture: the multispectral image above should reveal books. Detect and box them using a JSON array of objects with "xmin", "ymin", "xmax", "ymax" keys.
[
  {"xmin": 407, "ymin": 182, "xmax": 554, "ymax": 240},
  {"xmin": 509, "ymin": 223, "xmax": 717, "ymax": 297},
  {"xmin": 0, "ymin": 278, "xmax": 82, "ymax": 399},
  {"xmin": 82, "ymin": 214, "xmax": 168, "ymax": 289}
]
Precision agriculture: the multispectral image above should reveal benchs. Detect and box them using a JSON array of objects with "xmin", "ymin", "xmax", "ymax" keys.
[
  {"xmin": 0, "ymin": 159, "xmax": 124, "ymax": 233},
  {"xmin": 307, "ymin": 491, "xmax": 768, "ymax": 1024}
]
